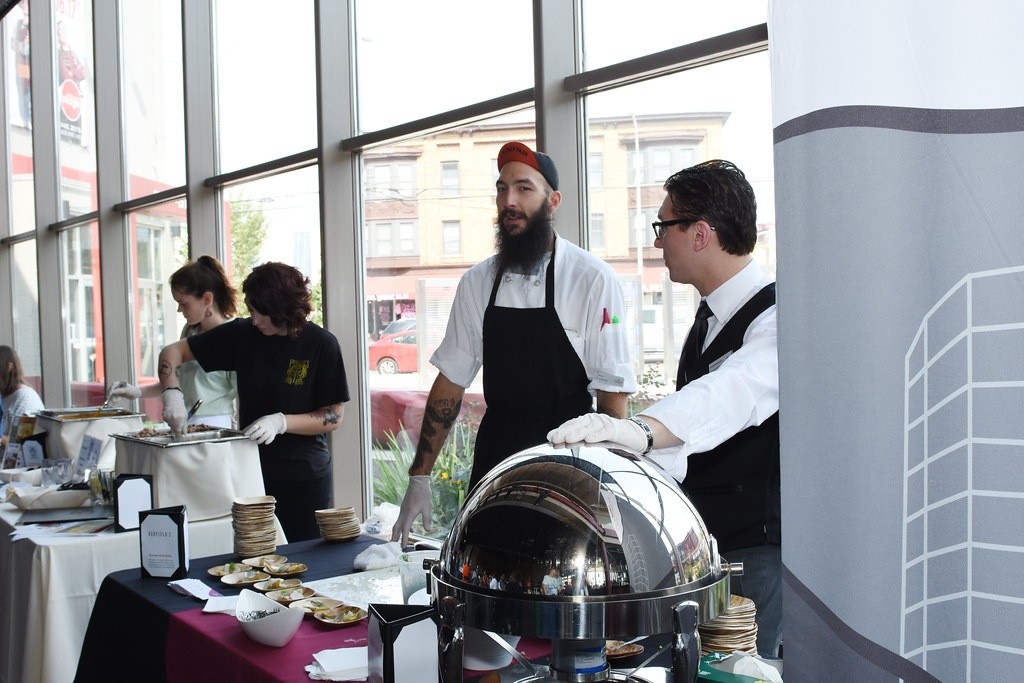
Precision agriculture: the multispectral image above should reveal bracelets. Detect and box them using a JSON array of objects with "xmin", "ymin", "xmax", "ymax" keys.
[{"xmin": 628, "ymin": 418, "xmax": 654, "ymax": 457}]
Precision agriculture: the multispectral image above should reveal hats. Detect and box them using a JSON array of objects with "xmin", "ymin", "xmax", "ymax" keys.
[{"xmin": 497, "ymin": 141, "xmax": 559, "ymax": 192}]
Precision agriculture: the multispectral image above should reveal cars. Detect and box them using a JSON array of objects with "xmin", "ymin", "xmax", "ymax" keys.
[
  {"xmin": 368, "ymin": 330, "xmax": 417, "ymax": 375},
  {"xmin": 379, "ymin": 318, "xmax": 416, "ymax": 339}
]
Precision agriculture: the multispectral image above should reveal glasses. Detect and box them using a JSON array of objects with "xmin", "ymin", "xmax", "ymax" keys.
[{"xmin": 652, "ymin": 219, "xmax": 716, "ymax": 238}]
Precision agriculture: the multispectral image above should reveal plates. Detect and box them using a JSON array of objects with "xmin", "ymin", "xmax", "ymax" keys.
[
  {"xmin": 207, "ymin": 496, "xmax": 370, "ymax": 649},
  {"xmin": 603, "ymin": 594, "xmax": 768, "ymax": 664}
]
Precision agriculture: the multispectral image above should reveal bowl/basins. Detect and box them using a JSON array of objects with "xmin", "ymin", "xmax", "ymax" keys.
[{"xmin": 0, "ymin": 456, "xmax": 117, "ymax": 508}]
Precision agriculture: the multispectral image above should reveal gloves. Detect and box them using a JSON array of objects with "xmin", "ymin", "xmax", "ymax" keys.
[
  {"xmin": 162, "ymin": 388, "xmax": 189, "ymax": 438},
  {"xmin": 243, "ymin": 412, "xmax": 286, "ymax": 444},
  {"xmin": 546, "ymin": 412, "xmax": 647, "ymax": 455},
  {"xmin": 390, "ymin": 474, "xmax": 432, "ymax": 549}
]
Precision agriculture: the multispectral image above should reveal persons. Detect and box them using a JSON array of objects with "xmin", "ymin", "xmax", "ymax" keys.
[
  {"xmin": 0, "ymin": 344, "xmax": 46, "ymax": 463},
  {"xmin": 158, "ymin": 261, "xmax": 351, "ymax": 543},
  {"xmin": 107, "ymin": 254, "xmax": 239, "ymax": 429},
  {"xmin": 449, "ymin": 554, "xmax": 592, "ymax": 598},
  {"xmin": 547, "ymin": 159, "xmax": 781, "ymax": 659},
  {"xmin": 11, "ymin": 0, "xmax": 31, "ymax": 129},
  {"xmin": 57, "ymin": 20, "xmax": 88, "ymax": 146},
  {"xmin": 392, "ymin": 141, "xmax": 636, "ymax": 549}
]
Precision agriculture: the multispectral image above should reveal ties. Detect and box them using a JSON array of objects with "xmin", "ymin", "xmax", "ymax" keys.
[{"xmin": 682, "ymin": 300, "xmax": 714, "ymax": 382}]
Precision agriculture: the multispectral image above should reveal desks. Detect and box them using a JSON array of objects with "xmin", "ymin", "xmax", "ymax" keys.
[
  {"xmin": 71, "ymin": 534, "xmax": 556, "ymax": 683},
  {"xmin": 0, "ymin": 454, "xmax": 287, "ymax": 683}
]
